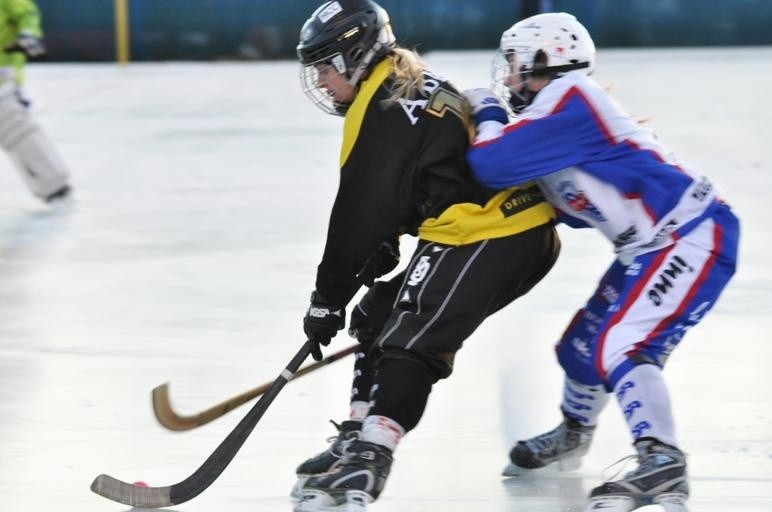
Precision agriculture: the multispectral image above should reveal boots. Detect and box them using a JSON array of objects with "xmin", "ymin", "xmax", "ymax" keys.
[
  {"xmin": 510, "ymin": 405, "xmax": 596, "ymax": 469},
  {"xmin": 589, "ymin": 436, "xmax": 688, "ymax": 511},
  {"xmin": 296, "ymin": 420, "xmax": 393, "ymax": 505}
]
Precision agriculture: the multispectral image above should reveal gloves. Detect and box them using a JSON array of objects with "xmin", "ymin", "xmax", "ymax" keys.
[
  {"xmin": 304, "ymin": 290, "xmax": 345, "ymax": 361},
  {"xmin": 363, "ymin": 231, "xmax": 400, "ymax": 288},
  {"xmin": 2, "ymin": 34, "xmax": 49, "ymax": 62},
  {"xmin": 460, "ymin": 87, "xmax": 508, "ymax": 124}
]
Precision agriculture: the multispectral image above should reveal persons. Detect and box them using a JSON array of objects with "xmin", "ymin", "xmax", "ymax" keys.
[
  {"xmin": 290, "ymin": 1, "xmax": 562, "ymax": 508},
  {"xmin": 461, "ymin": 9, "xmax": 743, "ymax": 510},
  {"xmin": 1, "ymin": 0, "xmax": 73, "ymax": 206}
]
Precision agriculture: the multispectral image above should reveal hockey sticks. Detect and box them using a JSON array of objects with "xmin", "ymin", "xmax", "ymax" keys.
[
  {"xmin": 90, "ymin": 340, "xmax": 312, "ymax": 508},
  {"xmin": 152, "ymin": 356, "xmax": 361, "ymax": 430}
]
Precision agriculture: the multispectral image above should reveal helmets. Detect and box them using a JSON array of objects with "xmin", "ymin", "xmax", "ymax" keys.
[
  {"xmin": 490, "ymin": 12, "xmax": 597, "ymax": 113},
  {"xmin": 295, "ymin": 0, "xmax": 398, "ymax": 117}
]
